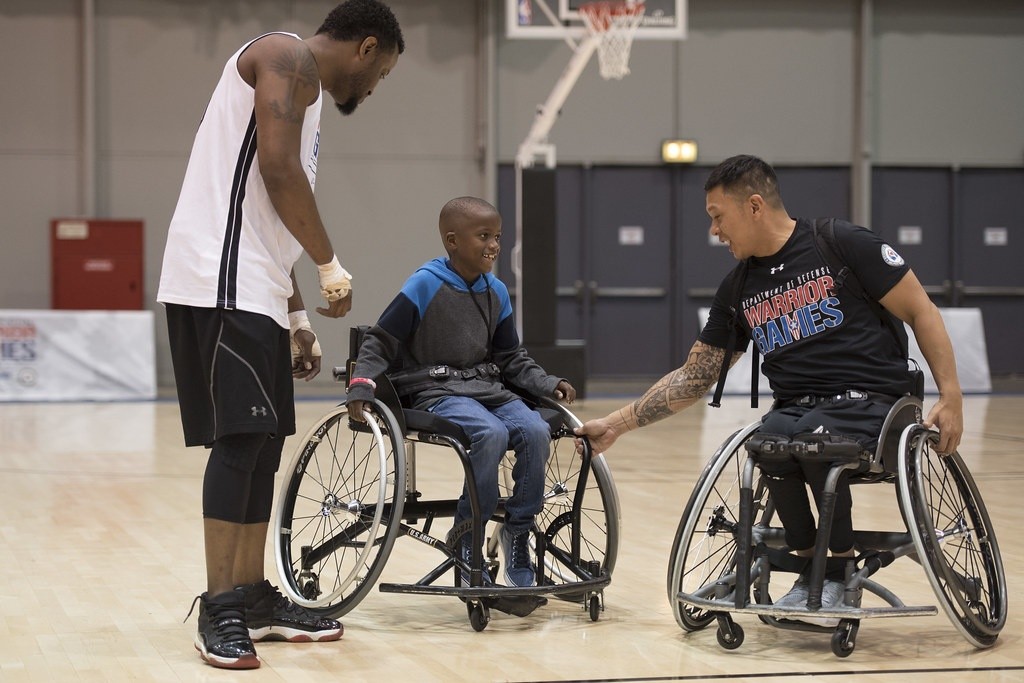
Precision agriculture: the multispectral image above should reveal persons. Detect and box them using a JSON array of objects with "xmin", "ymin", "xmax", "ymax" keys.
[
  {"xmin": 344, "ymin": 197, "xmax": 577, "ymax": 587},
  {"xmin": 574, "ymin": 154, "xmax": 963, "ymax": 608},
  {"xmin": 156, "ymin": 0, "xmax": 405, "ymax": 669}
]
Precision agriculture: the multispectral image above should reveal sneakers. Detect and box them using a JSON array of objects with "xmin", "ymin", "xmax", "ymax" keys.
[
  {"xmin": 234, "ymin": 579, "xmax": 343, "ymax": 642},
  {"xmin": 497, "ymin": 524, "xmax": 537, "ymax": 587},
  {"xmin": 461, "ymin": 540, "xmax": 492, "ymax": 588},
  {"xmin": 796, "ymin": 581, "xmax": 845, "ymax": 608},
  {"xmin": 774, "ymin": 573, "xmax": 811, "ymax": 609},
  {"xmin": 184, "ymin": 591, "xmax": 261, "ymax": 669}
]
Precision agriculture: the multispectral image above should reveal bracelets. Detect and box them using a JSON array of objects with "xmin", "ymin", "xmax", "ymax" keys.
[{"xmin": 349, "ymin": 378, "xmax": 376, "ymax": 389}]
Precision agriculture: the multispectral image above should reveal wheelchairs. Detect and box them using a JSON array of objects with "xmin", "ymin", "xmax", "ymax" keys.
[
  {"xmin": 664, "ymin": 355, "xmax": 1008, "ymax": 660},
  {"xmin": 272, "ymin": 325, "xmax": 623, "ymax": 633}
]
